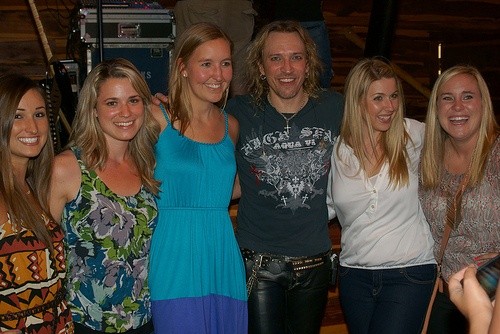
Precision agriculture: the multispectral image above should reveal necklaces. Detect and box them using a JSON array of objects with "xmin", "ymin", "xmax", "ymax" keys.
[{"xmin": 267, "ymin": 91, "xmax": 307, "ymax": 135}]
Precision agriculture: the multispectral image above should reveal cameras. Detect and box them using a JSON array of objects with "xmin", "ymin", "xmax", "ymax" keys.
[{"xmin": 460, "ymin": 252, "xmax": 500, "ymax": 302}]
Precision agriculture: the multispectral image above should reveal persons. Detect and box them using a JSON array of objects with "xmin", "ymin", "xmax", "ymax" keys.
[
  {"xmin": 418, "ymin": 66, "xmax": 500, "ymax": 334},
  {"xmin": 0, "ymin": 74, "xmax": 73, "ymax": 334},
  {"xmin": 147, "ymin": 23, "xmax": 249, "ymax": 334},
  {"xmin": 450, "ymin": 252, "xmax": 500, "ymax": 334},
  {"xmin": 326, "ymin": 59, "xmax": 441, "ymax": 334},
  {"xmin": 150, "ymin": 20, "xmax": 346, "ymax": 334},
  {"xmin": 47, "ymin": 57, "xmax": 155, "ymax": 334}
]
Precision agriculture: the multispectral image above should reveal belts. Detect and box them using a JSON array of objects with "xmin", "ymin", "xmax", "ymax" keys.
[{"xmin": 240, "ymin": 248, "xmax": 333, "ymax": 274}]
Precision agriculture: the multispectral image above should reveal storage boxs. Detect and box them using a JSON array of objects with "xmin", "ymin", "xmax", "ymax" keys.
[
  {"xmin": 77, "ymin": 43, "xmax": 175, "ymax": 97},
  {"xmin": 69, "ymin": 9, "xmax": 177, "ymax": 43}
]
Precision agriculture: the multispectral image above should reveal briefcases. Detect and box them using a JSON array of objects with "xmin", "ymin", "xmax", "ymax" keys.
[{"xmin": 69, "ymin": 0, "xmax": 178, "ymax": 49}]
